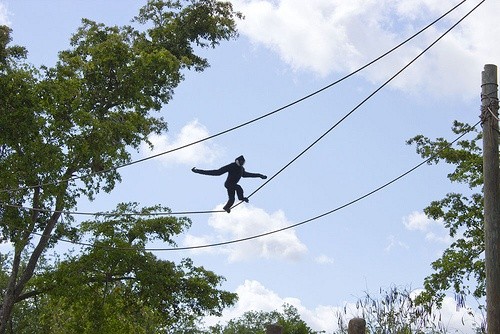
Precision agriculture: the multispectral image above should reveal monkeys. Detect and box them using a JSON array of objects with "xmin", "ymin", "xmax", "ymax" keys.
[{"xmin": 192, "ymin": 155, "xmax": 268, "ymax": 213}]
